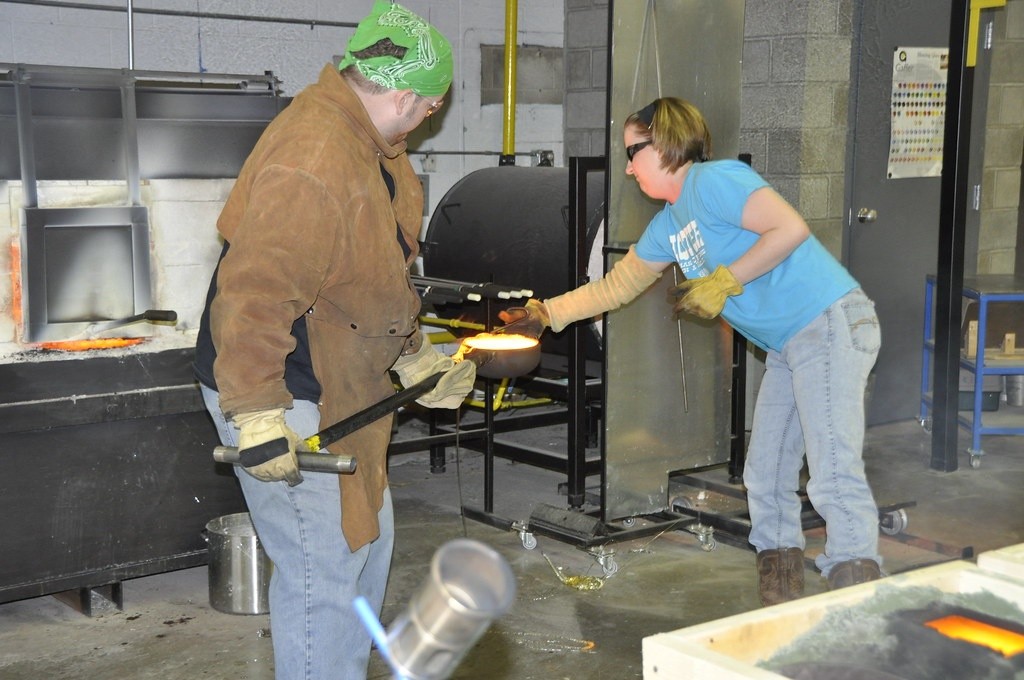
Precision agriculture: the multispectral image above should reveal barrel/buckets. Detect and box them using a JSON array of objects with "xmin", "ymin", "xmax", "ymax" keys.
[{"xmin": 200, "ymin": 510, "xmax": 272, "ymax": 616}]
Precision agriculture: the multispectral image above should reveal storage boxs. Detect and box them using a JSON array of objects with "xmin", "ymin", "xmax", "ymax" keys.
[{"xmin": 642, "ymin": 539, "xmax": 1024, "ymax": 680}]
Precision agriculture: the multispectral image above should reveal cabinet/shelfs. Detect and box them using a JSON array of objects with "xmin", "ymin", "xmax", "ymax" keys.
[{"xmin": 922, "ymin": 275, "xmax": 1024, "ymax": 456}]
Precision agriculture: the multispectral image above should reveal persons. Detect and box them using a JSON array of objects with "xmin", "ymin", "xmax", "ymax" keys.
[
  {"xmin": 491, "ymin": 96, "xmax": 885, "ymax": 609},
  {"xmin": 190, "ymin": 1, "xmax": 477, "ymax": 679}
]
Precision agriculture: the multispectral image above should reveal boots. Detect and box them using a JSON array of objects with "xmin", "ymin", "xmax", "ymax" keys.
[
  {"xmin": 757, "ymin": 547, "xmax": 804, "ymax": 608},
  {"xmin": 827, "ymin": 559, "xmax": 881, "ymax": 591}
]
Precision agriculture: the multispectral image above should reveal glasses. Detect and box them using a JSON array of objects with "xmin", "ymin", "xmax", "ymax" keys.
[
  {"xmin": 418, "ymin": 95, "xmax": 444, "ymax": 117},
  {"xmin": 627, "ymin": 140, "xmax": 653, "ymax": 162}
]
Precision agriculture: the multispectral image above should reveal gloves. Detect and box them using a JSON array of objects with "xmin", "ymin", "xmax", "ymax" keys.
[
  {"xmin": 667, "ymin": 265, "xmax": 743, "ymax": 320},
  {"xmin": 499, "ymin": 299, "xmax": 550, "ymax": 339},
  {"xmin": 231, "ymin": 408, "xmax": 311, "ymax": 486},
  {"xmin": 389, "ymin": 333, "xmax": 476, "ymax": 410}
]
